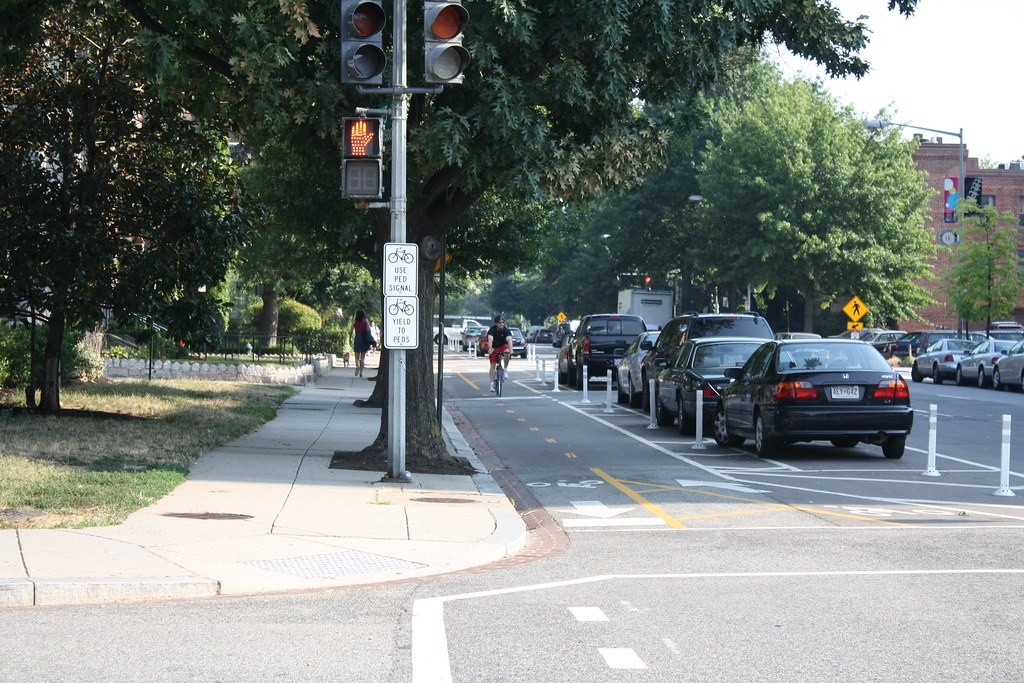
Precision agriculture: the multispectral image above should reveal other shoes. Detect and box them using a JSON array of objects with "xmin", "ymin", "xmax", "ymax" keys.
[
  {"xmin": 360, "ymin": 374, "xmax": 362, "ymax": 377},
  {"xmin": 503, "ymin": 370, "xmax": 508, "ymax": 379},
  {"xmin": 490, "ymin": 383, "xmax": 495, "ymax": 392},
  {"xmin": 355, "ymin": 366, "xmax": 359, "ymax": 377}
]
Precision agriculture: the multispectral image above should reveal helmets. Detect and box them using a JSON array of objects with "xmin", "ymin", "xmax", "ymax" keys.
[{"xmin": 494, "ymin": 316, "xmax": 505, "ymax": 323}]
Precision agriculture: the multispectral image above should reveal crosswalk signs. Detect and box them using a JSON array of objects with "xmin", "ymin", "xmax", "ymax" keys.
[{"xmin": 842, "ymin": 295, "xmax": 869, "ymax": 322}]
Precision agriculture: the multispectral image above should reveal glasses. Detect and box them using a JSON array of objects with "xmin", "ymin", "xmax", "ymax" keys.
[{"xmin": 498, "ymin": 322, "xmax": 504, "ymax": 324}]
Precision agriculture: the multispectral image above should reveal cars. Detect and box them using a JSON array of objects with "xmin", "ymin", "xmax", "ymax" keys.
[
  {"xmin": 911, "ymin": 338, "xmax": 977, "ymax": 384},
  {"xmin": 653, "ymin": 338, "xmax": 771, "ymax": 437},
  {"xmin": 552, "ymin": 313, "xmax": 647, "ymax": 390},
  {"xmin": 827, "ymin": 321, "xmax": 1024, "ymax": 358},
  {"xmin": 955, "ymin": 340, "xmax": 1018, "ymax": 388},
  {"xmin": 714, "ymin": 339, "xmax": 914, "ymax": 459},
  {"xmin": 992, "ymin": 340, "xmax": 1024, "ymax": 391},
  {"xmin": 613, "ymin": 330, "xmax": 663, "ymax": 409},
  {"xmin": 775, "ymin": 332, "xmax": 822, "ymax": 340},
  {"xmin": 525, "ymin": 326, "xmax": 553, "ymax": 342}
]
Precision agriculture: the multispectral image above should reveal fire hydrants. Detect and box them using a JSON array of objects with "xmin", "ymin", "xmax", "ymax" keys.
[{"xmin": 245, "ymin": 343, "xmax": 253, "ymax": 354}]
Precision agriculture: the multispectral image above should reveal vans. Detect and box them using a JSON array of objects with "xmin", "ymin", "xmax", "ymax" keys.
[{"xmin": 461, "ymin": 327, "xmax": 528, "ymax": 360}]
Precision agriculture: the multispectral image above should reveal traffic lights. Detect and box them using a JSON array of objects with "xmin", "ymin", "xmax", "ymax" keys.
[
  {"xmin": 424, "ymin": 1, "xmax": 471, "ymax": 84},
  {"xmin": 340, "ymin": 0, "xmax": 388, "ymax": 85},
  {"xmin": 340, "ymin": 117, "xmax": 384, "ymax": 202}
]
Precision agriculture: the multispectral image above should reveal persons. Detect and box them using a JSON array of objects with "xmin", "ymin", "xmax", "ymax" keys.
[
  {"xmin": 486, "ymin": 315, "xmax": 514, "ymax": 392},
  {"xmin": 370, "ymin": 321, "xmax": 380, "ymax": 355},
  {"xmin": 348, "ymin": 310, "xmax": 371, "ymax": 377}
]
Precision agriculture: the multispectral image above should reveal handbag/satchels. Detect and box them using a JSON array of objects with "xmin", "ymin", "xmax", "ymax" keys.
[{"xmin": 364, "ymin": 320, "xmax": 376, "ymax": 346}]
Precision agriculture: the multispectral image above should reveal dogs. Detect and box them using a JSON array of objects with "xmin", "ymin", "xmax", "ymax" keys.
[{"xmin": 343, "ymin": 353, "xmax": 349, "ymax": 367}]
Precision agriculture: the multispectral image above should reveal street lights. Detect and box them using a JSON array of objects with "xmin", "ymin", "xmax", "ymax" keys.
[
  {"xmin": 689, "ymin": 196, "xmax": 750, "ymax": 312},
  {"xmin": 864, "ymin": 120, "xmax": 963, "ymax": 339}
]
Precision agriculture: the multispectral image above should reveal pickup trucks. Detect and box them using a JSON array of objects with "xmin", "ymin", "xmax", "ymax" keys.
[{"xmin": 433, "ymin": 319, "xmax": 490, "ymax": 345}]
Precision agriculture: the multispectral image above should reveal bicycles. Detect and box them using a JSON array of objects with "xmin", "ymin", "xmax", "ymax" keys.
[{"xmin": 493, "ymin": 352, "xmax": 512, "ymax": 397}]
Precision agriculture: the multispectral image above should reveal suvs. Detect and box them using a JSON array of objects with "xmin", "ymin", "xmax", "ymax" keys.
[{"xmin": 640, "ymin": 311, "xmax": 775, "ymax": 426}]
